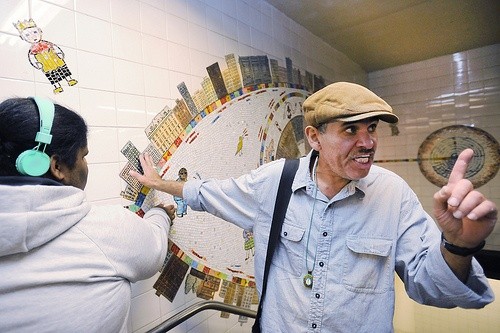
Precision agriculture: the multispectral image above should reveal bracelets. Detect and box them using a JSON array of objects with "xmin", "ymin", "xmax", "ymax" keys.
[{"xmin": 441, "ymin": 232, "xmax": 485, "ymax": 256}]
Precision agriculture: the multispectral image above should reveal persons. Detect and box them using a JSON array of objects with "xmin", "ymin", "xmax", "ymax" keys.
[
  {"xmin": 0, "ymin": 97, "xmax": 177, "ymax": 333},
  {"xmin": 129, "ymin": 82, "xmax": 497, "ymax": 333}
]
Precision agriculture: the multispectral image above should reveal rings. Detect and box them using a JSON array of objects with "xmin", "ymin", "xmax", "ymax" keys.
[{"xmin": 153, "ymin": 166, "xmax": 157, "ymax": 168}]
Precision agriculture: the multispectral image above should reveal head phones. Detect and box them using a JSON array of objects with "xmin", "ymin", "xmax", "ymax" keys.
[{"xmin": 15, "ymin": 95, "xmax": 55, "ymax": 177}]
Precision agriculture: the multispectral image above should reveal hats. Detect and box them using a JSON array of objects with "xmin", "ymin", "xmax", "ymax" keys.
[{"xmin": 302, "ymin": 81, "xmax": 400, "ymax": 127}]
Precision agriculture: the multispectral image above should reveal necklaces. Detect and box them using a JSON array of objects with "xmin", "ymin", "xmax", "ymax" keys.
[{"xmin": 303, "ymin": 172, "xmax": 318, "ymax": 287}]
